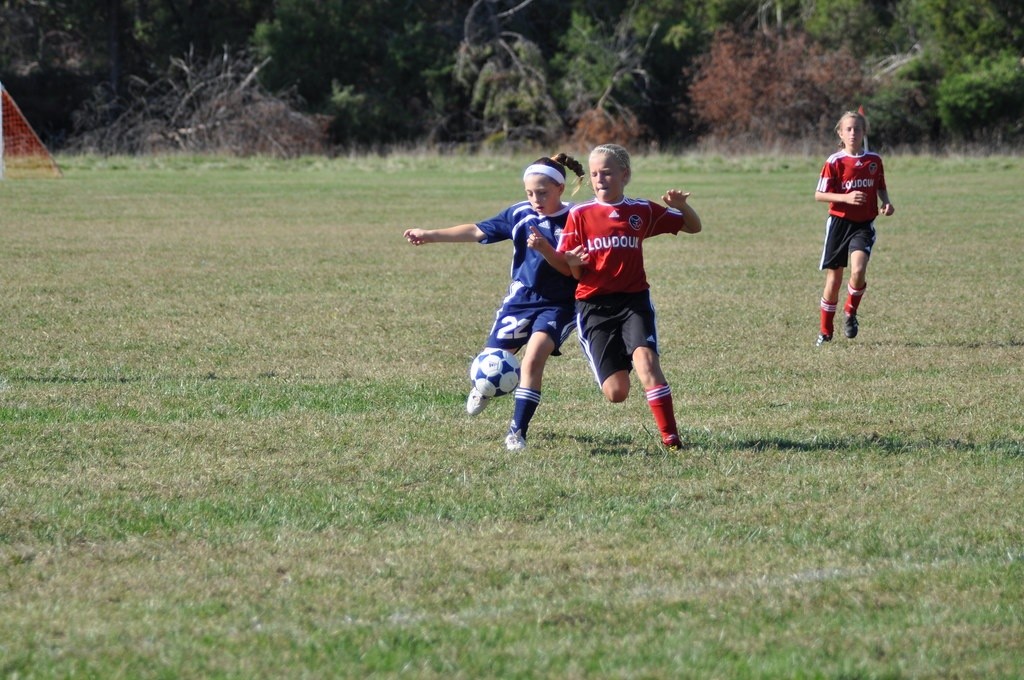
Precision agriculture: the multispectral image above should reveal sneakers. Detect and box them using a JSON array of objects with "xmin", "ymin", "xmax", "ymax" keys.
[
  {"xmin": 466, "ymin": 386, "xmax": 489, "ymax": 416},
  {"xmin": 842, "ymin": 308, "xmax": 859, "ymax": 339},
  {"xmin": 816, "ymin": 330, "xmax": 833, "ymax": 347},
  {"xmin": 504, "ymin": 430, "xmax": 527, "ymax": 452}
]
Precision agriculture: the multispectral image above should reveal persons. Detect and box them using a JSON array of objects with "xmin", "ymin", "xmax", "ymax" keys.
[
  {"xmin": 399, "ymin": 153, "xmax": 592, "ymax": 451},
  {"xmin": 526, "ymin": 142, "xmax": 703, "ymax": 452},
  {"xmin": 811, "ymin": 112, "xmax": 896, "ymax": 345}
]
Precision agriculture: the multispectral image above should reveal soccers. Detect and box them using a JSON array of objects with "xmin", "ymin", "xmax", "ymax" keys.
[{"xmin": 470, "ymin": 348, "xmax": 520, "ymax": 398}]
